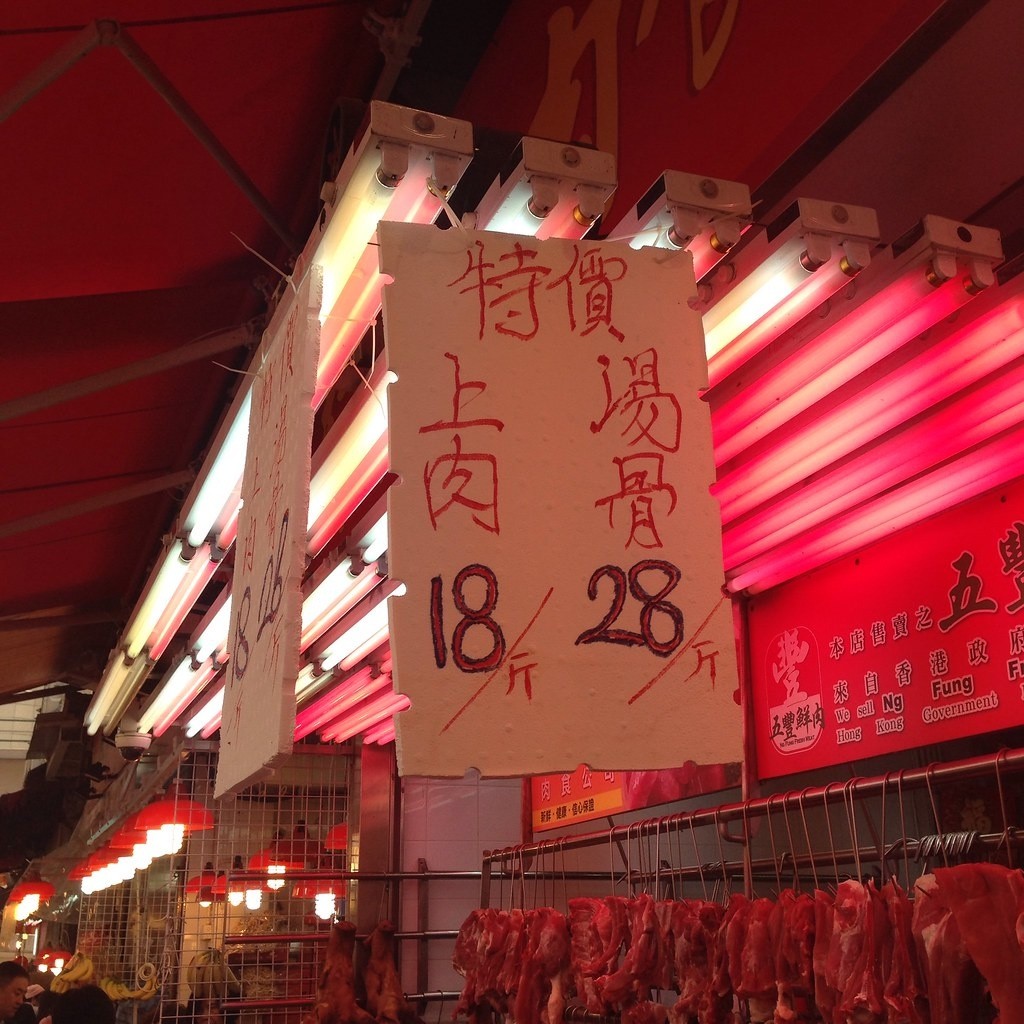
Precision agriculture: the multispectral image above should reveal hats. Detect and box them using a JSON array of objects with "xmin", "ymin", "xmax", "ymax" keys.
[
  {"xmin": 25, "ymin": 984, "xmax": 44, "ymax": 999},
  {"xmin": 48, "ymin": 984, "xmax": 117, "ymax": 1024}
]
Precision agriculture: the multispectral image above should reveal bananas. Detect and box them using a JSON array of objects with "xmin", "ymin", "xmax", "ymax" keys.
[
  {"xmin": 186, "ymin": 948, "xmax": 242, "ymax": 999},
  {"xmin": 49, "ymin": 951, "xmax": 161, "ymax": 1001}
]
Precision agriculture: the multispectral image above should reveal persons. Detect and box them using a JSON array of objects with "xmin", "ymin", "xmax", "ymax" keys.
[
  {"xmin": 186, "ymin": 992, "xmax": 239, "ymax": 1024},
  {"xmin": 26, "ymin": 983, "xmax": 116, "ymax": 1024},
  {"xmin": 0, "ymin": 961, "xmax": 38, "ymax": 1024}
]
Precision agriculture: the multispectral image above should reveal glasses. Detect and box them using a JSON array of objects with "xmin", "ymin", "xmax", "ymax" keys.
[{"xmin": 34, "ymin": 997, "xmax": 43, "ymax": 1000}]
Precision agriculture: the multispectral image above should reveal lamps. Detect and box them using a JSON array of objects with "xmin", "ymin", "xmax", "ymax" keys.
[
  {"xmin": 182, "ymin": 741, "xmax": 349, "ymax": 920},
  {"xmin": 6, "ymin": 874, "xmax": 55, "ymax": 920},
  {"xmin": 31, "ymin": 945, "xmax": 72, "ymax": 978},
  {"xmin": 82, "ymin": 96, "xmax": 1024, "ymax": 746},
  {"xmin": 66, "ymin": 776, "xmax": 214, "ymax": 895}
]
